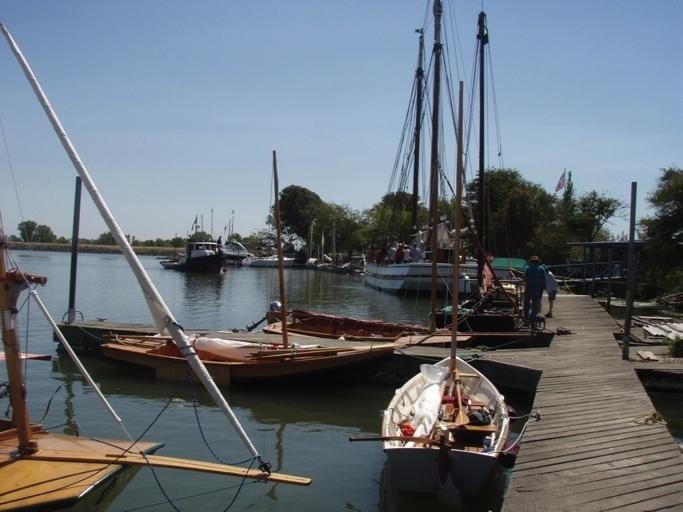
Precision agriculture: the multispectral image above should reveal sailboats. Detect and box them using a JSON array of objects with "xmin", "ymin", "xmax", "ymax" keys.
[
  {"xmin": 381, "ymin": 81, "xmax": 510, "ymax": 494},
  {"xmin": 1, "ymin": 22, "xmax": 311, "ymax": 512}
]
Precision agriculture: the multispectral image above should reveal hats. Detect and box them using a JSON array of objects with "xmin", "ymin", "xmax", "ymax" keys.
[{"xmin": 527, "ymin": 255, "xmax": 541, "ymax": 264}]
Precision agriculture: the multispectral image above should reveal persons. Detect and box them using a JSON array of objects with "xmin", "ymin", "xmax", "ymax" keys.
[
  {"xmin": 522, "ymin": 255, "xmax": 559, "ymax": 320},
  {"xmin": 334, "ymin": 238, "xmax": 424, "ymax": 267},
  {"xmin": 216, "ymin": 235, "xmax": 224, "ymax": 251},
  {"xmin": 219, "ymin": 265, "xmax": 229, "ymax": 275}
]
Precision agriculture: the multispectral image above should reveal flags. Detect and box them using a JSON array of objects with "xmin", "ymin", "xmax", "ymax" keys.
[{"xmin": 555, "ymin": 167, "xmax": 568, "ymax": 192}]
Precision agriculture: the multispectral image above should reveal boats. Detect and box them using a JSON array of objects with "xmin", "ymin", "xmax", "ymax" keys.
[
  {"xmin": 153, "ymin": 0, "xmax": 557, "ymax": 350},
  {"xmin": 99, "ymin": 151, "xmax": 408, "ymax": 389}
]
